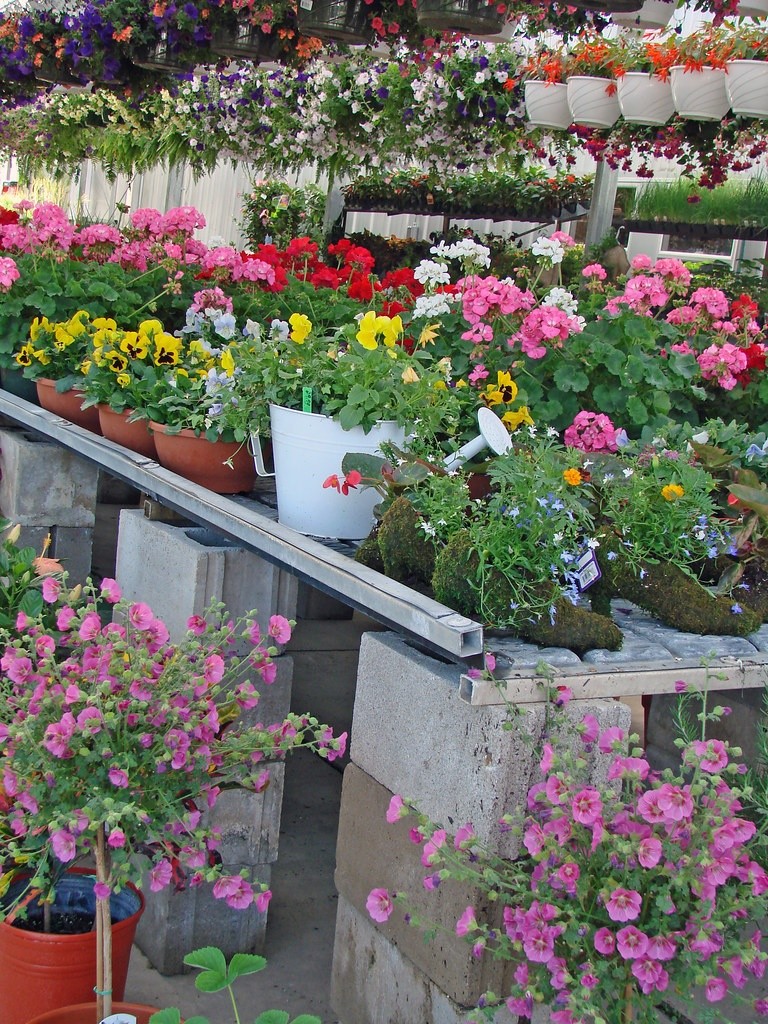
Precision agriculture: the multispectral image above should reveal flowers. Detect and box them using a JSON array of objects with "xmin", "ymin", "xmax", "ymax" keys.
[{"xmin": 0, "ymin": 1, "xmax": 768, "ymax": 1023}]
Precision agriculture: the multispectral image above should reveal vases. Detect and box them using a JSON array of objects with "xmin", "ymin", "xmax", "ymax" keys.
[
  {"xmin": 0, "ymin": 863, "xmax": 145, "ymax": 1024},
  {"xmin": 100, "ymin": 60, "xmax": 128, "ymax": 84},
  {"xmin": 555, "ymin": 0, "xmax": 644, "ymax": 11},
  {"xmin": 465, "ymin": 471, "xmax": 494, "ymax": 517},
  {"xmin": 616, "ymin": 71, "xmax": 675, "ymax": 126},
  {"xmin": 565, "ymin": 75, "xmax": 622, "ymax": 128},
  {"xmin": 737, "ymin": 225, "xmax": 752, "ymax": 239},
  {"xmin": 33, "ymin": 55, "xmax": 60, "ymax": 82},
  {"xmin": 659, "ymin": 221, "xmax": 674, "ymax": 234},
  {"xmin": 737, "ymin": 0, "xmax": 768, "ymax": 16},
  {"xmin": 32, "ymin": 375, "xmax": 103, "ymax": 435},
  {"xmin": 0, "ymin": 368, "xmax": 41, "ymax": 406},
  {"xmin": 720, "ymin": 225, "xmax": 735, "ymax": 238},
  {"xmin": 648, "ymin": 221, "xmax": 661, "ymax": 232},
  {"xmin": 55, "ymin": 65, "xmax": 85, "ymax": 87},
  {"xmin": 126, "ymin": 63, "xmax": 150, "ymax": 83},
  {"xmin": 296, "ymin": 0, "xmax": 383, "ymax": 44},
  {"xmin": 750, "ymin": 226, "xmax": 767, "ymax": 240},
  {"xmin": 675, "ymin": 221, "xmax": 692, "ymax": 236},
  {"xmin": 637, "ymin": 220, "xmax": 650, "ymax": 232},
  {"xmin": 668, "ymin": 64, "xmax": 730, "ymax": 121},
  {"xmin": 551, "ymin": 198, "xmax": 590, "ymax": 221},
  {"xmin": 130, "ymin": 49, "xmax": 159, "ymax": 71},
  {"xmin": 25, "ymin": 1001, "xmax": 186, "ymax": 1024},
  {"xmin": 466, "ymin": 14, "xmax": 522, "ymax": 43},
  {"xmin": 149, "ymin": 418, "xmax": 272, "ymax": 494},
  {"xmin": 152, "ymin": 27, "xmax": 199, "ymax": 74},
  {"xmin": 523, "ymin": 79, "xmax": 573, "ymax": 130},
  {"xmin": 689, "ymin": 223, "xmax": 706, "ymax": 238},
  {"xmin": 207, "ymin": 18, "xmax": 289, "ymax": 60},
  {"xmin": 68, "ymin": 56, "xmax": 96, "ymax": 74},
  {"xmin": 623, "ymin": 219, "xmax": 639, "ymax": 231},
  {"xmin": 414, "ymin": 1, "xmax": 509, "ymax": 35},
  {"xmin": 541, "ymin": 17, "xmax": 564, "ymax": 30},
  {"xmin": 724, "ymin": 58, "xmax": 768, "ymax": 119},
  {"xmin": 94, "ymin": 401, "xmax": 158, "ymax": 458},
  {"xmin": 703, "ymin": 224, "xmax": 719, "ymax": 238},
  {"xmin": 611, "ymin": 0, "xmax": 676, "ymax": 28}
]
